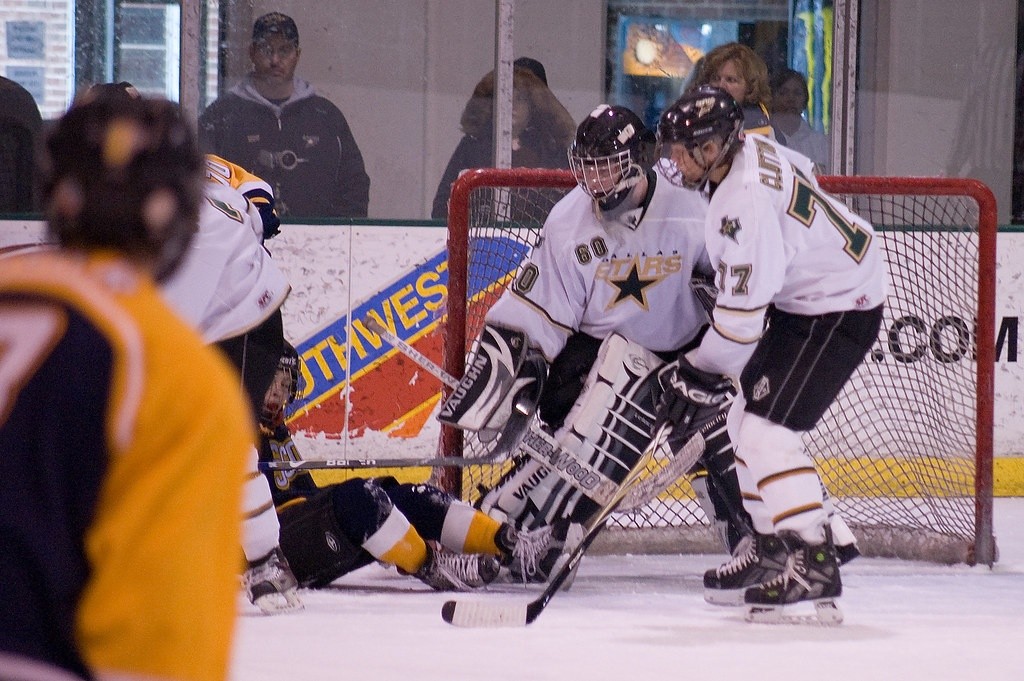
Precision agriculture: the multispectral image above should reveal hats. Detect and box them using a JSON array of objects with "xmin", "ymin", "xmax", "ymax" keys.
[{"xmin": 256, "ymin": 12, "xmax": 299, "ymax": 42}]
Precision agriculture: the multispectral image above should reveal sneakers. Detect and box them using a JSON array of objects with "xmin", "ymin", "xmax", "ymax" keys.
[
  {"xmin": 744, "ymin": 523, "xmax": 844, "ymax": 626},
  {"xmin": 704, "ymin": 533, "xmax": 788, "ymax": 619},
  {"xmin": 397, "ymin": 535, "xmax": 509, "ymax": 593},
  {"xmin": 494, "ymin": 517, "xmax": 588, "ymax": 593}
]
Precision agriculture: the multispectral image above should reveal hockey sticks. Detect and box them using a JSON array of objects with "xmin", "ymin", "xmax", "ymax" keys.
[
  {"xmin": 442, "ymin": 419, "xmax": 674, "ymax": 629},
  {"xmin": 257, "ymin": 354, "xmax": 546, "ymax": 470},
  {"xmin": 361, "ymin": 314, "xmax": 706, "ymax": 513}
]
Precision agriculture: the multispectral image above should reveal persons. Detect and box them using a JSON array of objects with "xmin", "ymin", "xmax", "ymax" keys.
[
  {"xmin": 435, "ymin": 103, "xmax": 862, "ymax": 584},
  {"xmin": 432, "ymin": 41, "xmax": 831, "ymax": 225},
  {"xmin": 0, "ymin": 12, "xmax": 371, "ymax": 219},
  {"xmin": 0, "ymin": 94, "xmax": 252, "ymax": 681},
  {"xmin": 78, "ymin": 80, "xmax": 569, "ymax": 605},
  {"xmin": 655, "ymin": 84, "xmax": 887, "ymax": 610}
]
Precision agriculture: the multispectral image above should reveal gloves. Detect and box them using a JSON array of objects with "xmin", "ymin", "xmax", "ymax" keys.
[
  {"xmin": 655, "ymin": 353, "xmax": 736, "ymax": 440},
  {"xmin": 247, "ymin": 544, "xmax": 306, "ymax": 615},
  {"xmin": 689, "ymin": 262, "xmax": 717, "ymax": 328},
  {"xmin": 255, "ymin": 205, "xmax": 281, "ymax": 240}
]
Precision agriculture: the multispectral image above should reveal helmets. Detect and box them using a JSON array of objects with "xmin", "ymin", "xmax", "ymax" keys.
[
  {"xmin": 652, "ymin": 83, "xmax": 747, "ymax": 191},
  {"xmin": 259, "ymin": 338, "xmax": 300, "ymax": 425},
  {"xmin": 570, "ymin": 103, "xmax": 657, "ymax": 221},
  {"xmin": 42, "ymin": 81, "xmax": 206, "ymax": 284}
]
